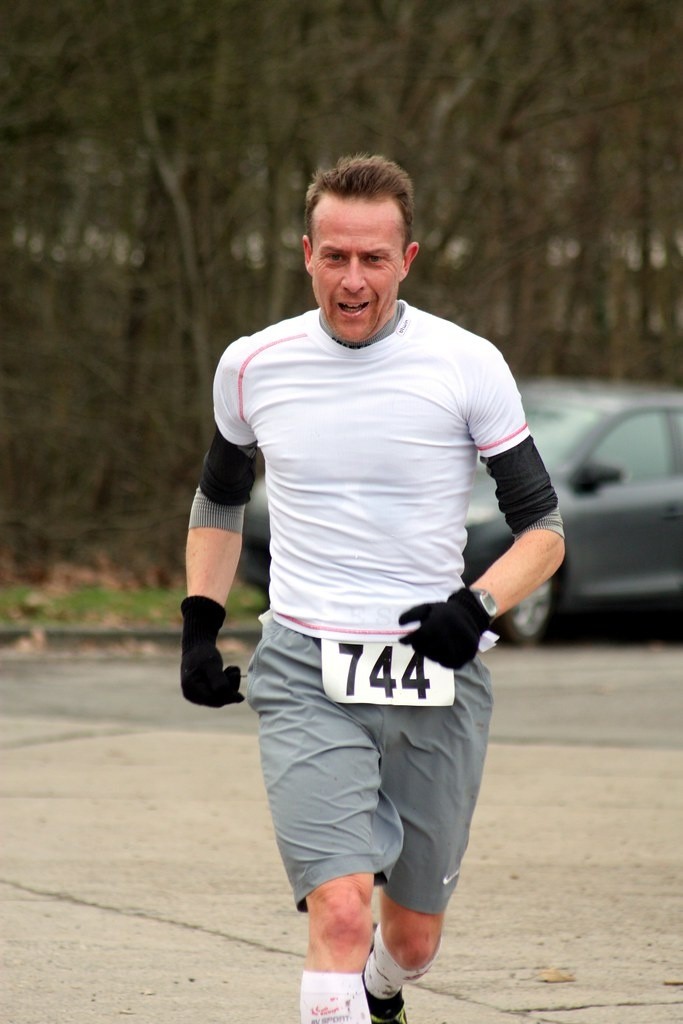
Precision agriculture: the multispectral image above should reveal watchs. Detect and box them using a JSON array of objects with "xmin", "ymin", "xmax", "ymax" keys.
[{"xmin": 469, "ymin": 586, "xmax": 498, "ymax": 627}]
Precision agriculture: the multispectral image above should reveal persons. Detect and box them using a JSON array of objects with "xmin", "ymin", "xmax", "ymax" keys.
[{"xmin": 180, "ymin": 158, "xmax": 564, "ymax": 1024}]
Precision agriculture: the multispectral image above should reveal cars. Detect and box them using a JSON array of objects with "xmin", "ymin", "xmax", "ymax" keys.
[{"xmin": 234, "ymin": 380, "xmax": 682, "ymax": 645}]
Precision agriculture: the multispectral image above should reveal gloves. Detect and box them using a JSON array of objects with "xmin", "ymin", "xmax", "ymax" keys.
[
  {"xmin": 399, "ymin": 587, "xmax": 489, "ymax": 671},
  {"xmin": 180, "ymin": 595, "xmax": 245, "ymax": 708}
]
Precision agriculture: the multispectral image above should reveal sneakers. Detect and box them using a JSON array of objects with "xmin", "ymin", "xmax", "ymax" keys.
[{"xmin": 363, "ymin": 946, "xmax": 409, "ymax": 1024}]
